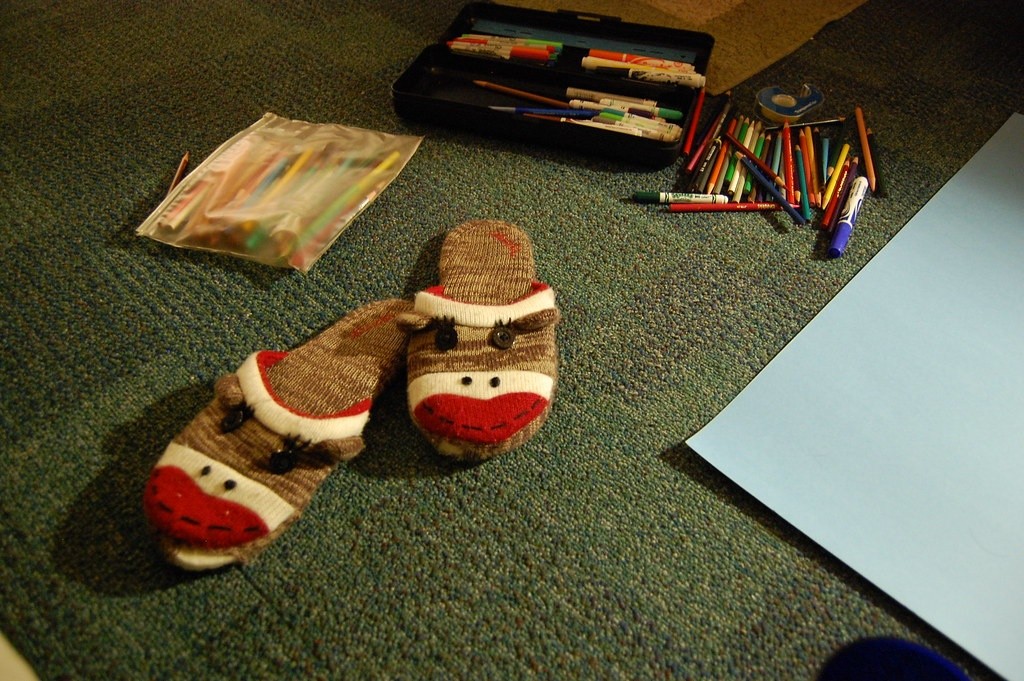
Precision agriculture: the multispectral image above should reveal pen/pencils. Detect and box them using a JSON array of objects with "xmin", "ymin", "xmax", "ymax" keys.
[
  {"xmin": 855, "ymin": 107, "xmax": 876, "ymax": 190},
  {"xmin": 167, "ymin": 151, "xmax": 190, "ymax": 198},
  {"xmin": 473, "ymin": 78, "xmax": 569, "ymax": 107},
  {"xmin": 687, "ymin": 90, "xmax": 859, "ymax": 235},
  {"xmin": 162, "ymin": 132, "xmax": 401, "ymax": 267},
  {"xmin": 683, "ymin": 85, "xmax": 706, "ymax": 154},
  {"xmin": 490, "ymin": 104, "xmax": 600, "ymax": 118},
  {"xmin": 669, "ymin": 203, "xmax": 802, "ymax": 211},
  {"xmin": 868, "ymin": 128, "xmax": 889, "ymax": 198}
]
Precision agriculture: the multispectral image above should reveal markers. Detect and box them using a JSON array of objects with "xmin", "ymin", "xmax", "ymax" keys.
[
  {"xmin": 566, "ymin": 86, "xmax": 685, "ymax": 142},
  {"xmin": 829, "ymin": 177, "xmax": 869, "ymax": 258},
  {"xmin": 558, "ymin": 45, "xmax": 706, "ymax": 89},
  {"xmin": 633, "ymin": 191, "xmax": 730, "ymax": 206},
  {"xmin": 446, "ymin": 33, "xmax": 562, "ymax": 59}
]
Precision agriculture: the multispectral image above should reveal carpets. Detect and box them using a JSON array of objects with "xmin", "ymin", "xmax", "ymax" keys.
[{"xmin": 495, "ymin": 0, "xmax": 866, "ymax": 95}]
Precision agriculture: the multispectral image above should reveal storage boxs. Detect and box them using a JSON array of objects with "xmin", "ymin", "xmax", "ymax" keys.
[{"xmin": 392, "ymin": 0, "xmax": 714, "ymax": 169}]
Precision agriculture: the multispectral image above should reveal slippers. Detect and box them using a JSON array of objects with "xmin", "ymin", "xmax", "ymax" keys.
[
  {"xmin": 144, "ymin": 298, "xmax": 414, "ymax": 573},
  {"xmin": 391, "ymin": 218, "xmax": 558, "ymax": 461}
]
